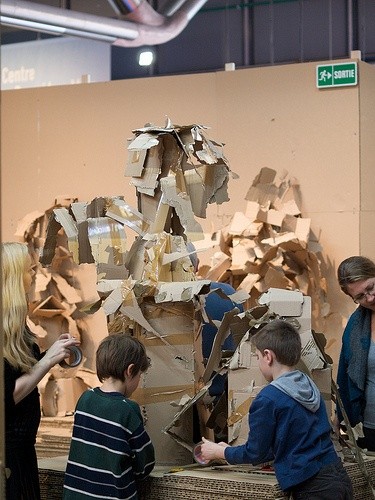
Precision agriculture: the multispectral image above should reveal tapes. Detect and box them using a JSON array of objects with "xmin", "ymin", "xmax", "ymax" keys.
[
  {"xmin": 58, "ymin": 344, "xmax": 83, "ymax": 369},
  {"xmin": 191, "ymin": 439, "xmax": 214, "ymax": 466}
]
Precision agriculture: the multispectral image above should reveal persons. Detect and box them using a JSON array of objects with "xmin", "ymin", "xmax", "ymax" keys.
[
  {"xmin": 187, "ymin": 242, "xmax": 247, "ymax": 441},
  {"xmin": 335, "ymin": 256, "xmax": 375, "ymax": 455},
  {"xmin": 0, "ymin": 241, "xmax": 82, "ymax": 499},
  {"xmin": 63, "ymin": 334, "xmax": 156, "ymax": 500},
  {"xmin": 200, "ymin": 321, "xmax": 353, "ymax": 500}
]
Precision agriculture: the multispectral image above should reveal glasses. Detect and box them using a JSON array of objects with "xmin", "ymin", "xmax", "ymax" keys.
[{"xmin": 354, "ymin": 287, "xmax": 375, "ymax": 304}]
